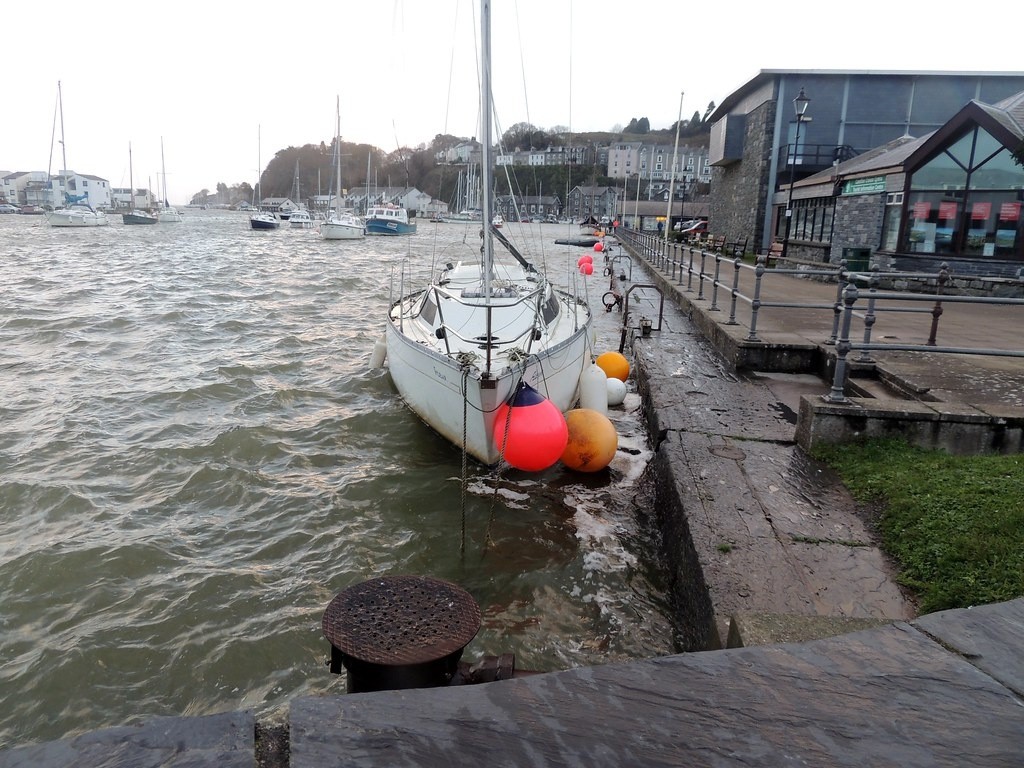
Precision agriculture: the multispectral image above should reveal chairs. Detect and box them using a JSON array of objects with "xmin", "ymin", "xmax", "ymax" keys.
[
  {"xmin": 753, "ymin": 238, "xmax": 787, "ymax": 268},
  {"xmin": 725, "ymin": 234, "xmax": 749, "ymax": 259},
  {"xmin": 688, "ymin": 232, "xmax": 726, "ymax": 253}
]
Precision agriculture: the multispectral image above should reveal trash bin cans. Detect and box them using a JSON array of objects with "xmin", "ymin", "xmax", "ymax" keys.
[{"xmin": 841, "ymin": 247, "xmax": 872, "ymax": 289}]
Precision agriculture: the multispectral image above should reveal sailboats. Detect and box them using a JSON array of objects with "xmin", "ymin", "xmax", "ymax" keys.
[
  {"xmin": 120, "ymin": 138, "xmax": 160, "ymax": 225},
  {"xmin": 518, "ymin": 180, "xmax": 614, "ymax": 238},
  {"xmin": 318, "ymin": 95, "xmax": 367, "ymax": 241},
  {"xmin": 381, "ymin": 0, "xmax": 634, "ymax": 477},
  {"xmin": 428, "ymin": 161, "xmax": 496, "ymax": 224},
  {"xmin": 155, "ymin": 136, "xmax": 183, "ymax": 222},
  {"xmin": 279, "ymin": 160, "xmax": 301, "ymax": 220},
  {"xmin": 249, "ymin": 124, "xmax": 280, "ymax": 230},
  {"xmin": 38, "ymin": 79, "xmax": 110, "ymax": 227}
]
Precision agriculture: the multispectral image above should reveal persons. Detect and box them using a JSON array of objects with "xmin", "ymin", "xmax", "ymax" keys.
[
  {"xmin": 354, "ymin": 201, "xmax": 364, "ymax": 216},
  {"xmin": 608, "ymin": 220, "xmax": 619, "ymax": 231},
  {"xmin": 657, "ymin": 220, "xmax": 663, "ymax": 237}
]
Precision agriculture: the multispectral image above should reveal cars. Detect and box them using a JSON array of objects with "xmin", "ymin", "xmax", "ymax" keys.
[
  {"xmin": 0, "ymin": 204, "xmax": 21, "ymax": 213},
  {"xmin": 674, "ymin": 220, "xmax": 708, "ymax": 235}
]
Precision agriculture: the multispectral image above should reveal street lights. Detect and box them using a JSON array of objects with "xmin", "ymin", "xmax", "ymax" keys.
[
  {"xmin": 779, "ymin": 85, "xmax": 811, "ymax": 258},
  {"xmin": 679, "ymin": 166, "xmax": 689, "ymax": 233}
]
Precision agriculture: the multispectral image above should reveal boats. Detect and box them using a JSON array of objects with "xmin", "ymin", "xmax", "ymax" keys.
[
  {"xmin": 491, "ymin": 215, "xmax": 504, "ymax": 228},
  {"xmin": 288, "ymin": 209, "xmax": 315, "ymax": 229},
  {"xmin": 363, "ymin": 203, "xmax": 418, "ymax": 236},
  {"xmin": 554, "ymin": 238, "xmax": 601, "ymax": 247}
]
